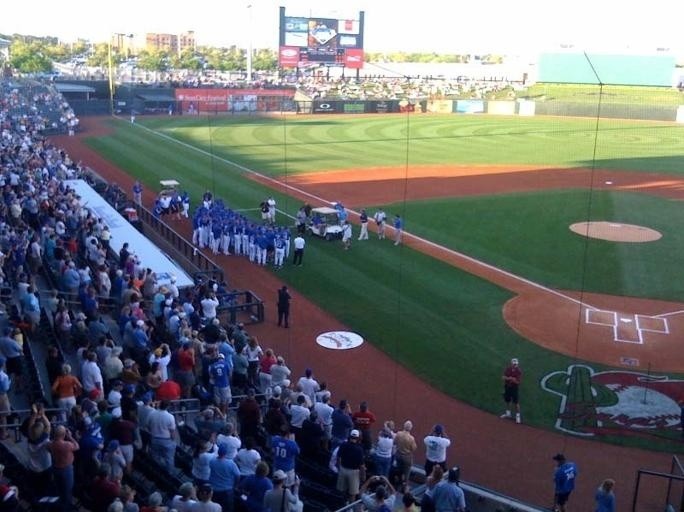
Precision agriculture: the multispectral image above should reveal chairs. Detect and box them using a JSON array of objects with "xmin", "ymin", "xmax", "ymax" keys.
[
  {"xmin": 123, "ymin": 72, "xmax": 515, "ymax": 108},
  {"xmin": 0, "ymin": 298, "xmax": 355, "ymax": 510},
  {"xmin": 0, "ymin": 74, "xmax": 122, "ymax": 298}
]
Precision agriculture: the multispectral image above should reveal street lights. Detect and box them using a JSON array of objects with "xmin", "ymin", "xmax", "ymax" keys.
[
  {"xmin": 177, "ymin": 29, "xmax": 195, "ymax": 60},
  {"xmin": 106, "ymin": 30, "xmax": 133, "ymax": 116},
  {"xmin": 244, "ymin": 3, "xmax": 253, "ymax": 80}
]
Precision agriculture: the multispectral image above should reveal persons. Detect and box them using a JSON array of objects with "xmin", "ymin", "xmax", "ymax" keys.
[
  {"xmin": 551, "ymin": 452, "xmax": 578, "ymax": 511},
  {"xmin": 593, "ymin": 477, "xmax": 617, "ymax": 512},
  {"xmin": 0, "ymin": 75, "xmax": 92, "ymax": 511},
  {"xmin": 499, "ymin": 357, "xmax": 521, "ymax": 423}
]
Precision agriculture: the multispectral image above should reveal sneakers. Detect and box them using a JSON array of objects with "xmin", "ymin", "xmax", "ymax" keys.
[
  {"xmin": 515, "ymin": 416, "xmax": 521, "ymax": 424},
  {"xmin": 500, "ymin": 413, "xmax": 512, "ymax": 419}
]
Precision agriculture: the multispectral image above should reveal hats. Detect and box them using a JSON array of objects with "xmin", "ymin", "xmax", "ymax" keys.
[
  {"xmin": 511, "ymin": 357, "xmax": 519, "ymax": 366},
  {"xmin": 432, "ymin": 424, "xmax": 445, "ymax": 434},
  {"xmin": 552, "ymin": 453, "xmax": 565, "ymax": 461},
  {"xmin": 305, "ymin": 368, "xmax": 312, "ymax": 375},
  {"xmin": 403, "ymin": 419, "xmax": 413, "ymax": 432},
  {"xmin": 448, "ymin": 465, "xmax": 459, "ymax": 483},
  {"xmin": 199, "ymin": 480, "xmax": 214, "ymax": 494},
  {"xmin": 350, "ymin": 429, "xmax": 360, "ymax": 439},
  {"xmin": 272, "ymin": 469, "xmax": 287, "ymax": 481},
  {"xmin": 3, "ymin": 253, "xmax": 292, "ymax": 457}
]
